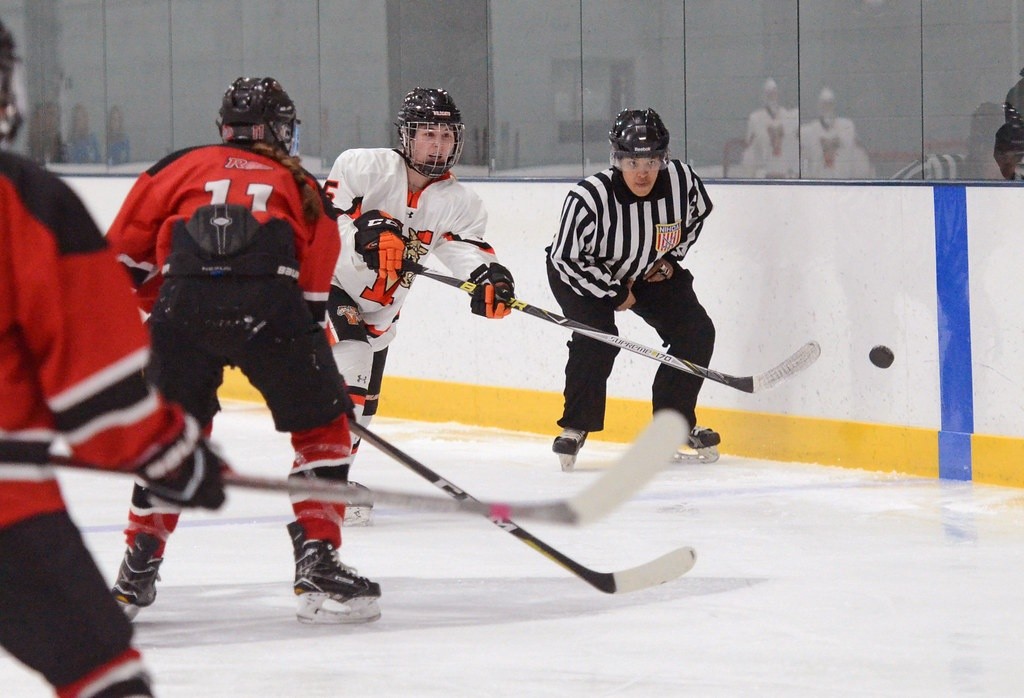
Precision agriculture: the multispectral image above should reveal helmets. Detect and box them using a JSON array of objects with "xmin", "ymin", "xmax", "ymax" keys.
[
  {"xmin": 215, "ymin": 77, "xmax": 301, "ymax": 157},
  {"xmin": 0, "ymin": 21, "xmax": 24, "ymax": 148},
  {"xmin": 609, "ymin": 107, "xmax": 670, "ymax": 160},
  {"xmin": 397, "ymin": 86, "xmax": 466, "ymax": 178}
]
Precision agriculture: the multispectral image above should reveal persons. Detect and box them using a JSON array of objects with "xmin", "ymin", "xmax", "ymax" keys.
[
  {"xmin": 102, "ymin": 77, "xmax": 381, "ymax": 626},
  {"xmin": 741, "ymin": 78, "xmax": 872, "ymax": 177},
  {"xmin": 888, "ymin": 102, "xmax": 1024, "ymax": 181},
  {"xmin": 0, "ymin": 21, "xmax": 233, "ymax": 698},
  {"xmin": 321, "ymin": 87, "xmax": 515, "ymax": 526},
  {"xmin": 544, "ymin": 108, "xmax": 721, "ymax": 468}
]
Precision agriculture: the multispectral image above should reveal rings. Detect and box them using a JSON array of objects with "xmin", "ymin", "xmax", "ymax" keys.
[{"xmin": 658, "ymin": 264, "xmax": 669, "ymax": 276}]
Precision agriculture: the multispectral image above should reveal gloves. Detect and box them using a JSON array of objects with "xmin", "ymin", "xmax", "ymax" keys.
[
  {"xmin": 138, "ymin": 403, "xmax": 233, "ymax": 509},
  {"xmin": 467, "ymin": 262, "xmax": 515, "ymax": 319},
  {"xmin": 353, "ymin": 209, "xmax": 407, "ymax": 279}
]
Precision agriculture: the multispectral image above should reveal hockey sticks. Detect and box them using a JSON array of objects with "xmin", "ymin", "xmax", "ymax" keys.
[
  {"xmin": 344, "ymin": 418, "xmax": 700, "ymax": 598},
  {"xmin": 45, "ymin": 405, "xmax": 696, "ymax": 532},
  {"xmin": 362, "ymin": 254, "xmax": 823, "ymax": 397}
]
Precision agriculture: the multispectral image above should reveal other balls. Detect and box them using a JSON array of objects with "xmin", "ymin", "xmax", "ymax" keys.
[{"xmin": 868, "ymin": 344, "xmax": 895, "ymax": 369}]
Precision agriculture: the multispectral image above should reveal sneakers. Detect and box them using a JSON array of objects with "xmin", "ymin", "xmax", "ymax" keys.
[
  {"xmin": 111, "ymin": 533, "xmax": 165, "ymax": 620},
  {"xmin": 669, "ymin": 426, "xmax": 720, "ymax": 463},
  {"xmin": 287, "ymin": 523, "xmax": 382, "ymax": 624},
  {"xmin": 553, "ymin": 427, "xmax": 588, "ymax": 472},
  {"xmin": 344, "ymin": 480, "xmax": 373, "ymax": 526}
]
